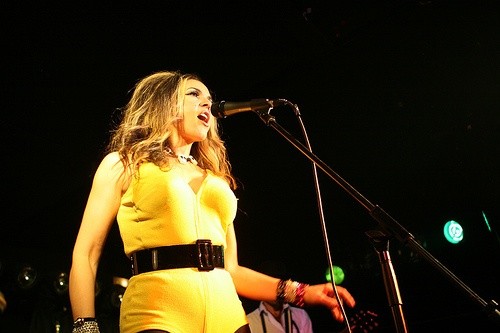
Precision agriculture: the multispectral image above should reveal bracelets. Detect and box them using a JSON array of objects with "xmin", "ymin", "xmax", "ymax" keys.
[
  {"xmin": 276, "ymin": 279, "xmax": 310, "ymax": 306},
  {"xmin": 72, "ymin": 317, "xmax": 100, "ymax": 333}
]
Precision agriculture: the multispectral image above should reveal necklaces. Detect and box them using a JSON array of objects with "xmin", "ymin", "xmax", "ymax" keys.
[{"xmin": 175, "ymin": 154, "xmax": 194, "ymax": 164}]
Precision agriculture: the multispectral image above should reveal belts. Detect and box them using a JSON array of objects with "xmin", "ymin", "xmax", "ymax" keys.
[{"xmin": 126, "ymin": 239, "xmax": 226, "ymax": 273}]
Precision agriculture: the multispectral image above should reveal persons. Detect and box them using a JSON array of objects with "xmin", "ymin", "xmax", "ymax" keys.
[
  {"xmin": 246, "ymin": 299, "xmax": 313, "ymax": 333},
  {"xmin": 69, "ymin": 71, "xmax": 355, "ymax": 333}
]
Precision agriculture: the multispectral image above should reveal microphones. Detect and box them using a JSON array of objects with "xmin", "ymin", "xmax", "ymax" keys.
[{"xmin": 210, "ymin": 98, "xmax": 276, "ymax": 118}]
